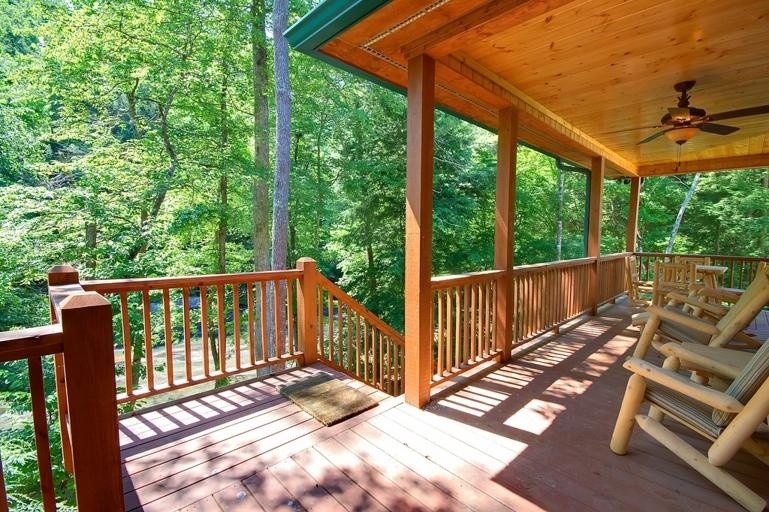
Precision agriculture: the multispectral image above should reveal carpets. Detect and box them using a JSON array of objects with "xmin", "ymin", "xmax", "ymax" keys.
[{"xmin": 275, "ymin": 369, "xmax": 379, "ymax": 429}]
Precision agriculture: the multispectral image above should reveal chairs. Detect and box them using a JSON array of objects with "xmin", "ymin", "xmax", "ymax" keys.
[{"xmin": 605, "ymin": 253, "xmax": 769, "ymax": 512}]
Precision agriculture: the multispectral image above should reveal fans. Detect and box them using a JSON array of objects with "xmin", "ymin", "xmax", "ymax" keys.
[{"xmin": 590, "ymin": 78, "xmax": 768, "ymax": 145}]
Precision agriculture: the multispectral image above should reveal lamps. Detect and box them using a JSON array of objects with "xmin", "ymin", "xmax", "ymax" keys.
[{"xmin": 662, "ymin": 128, "xmax": 701, "ymax": 145}]
